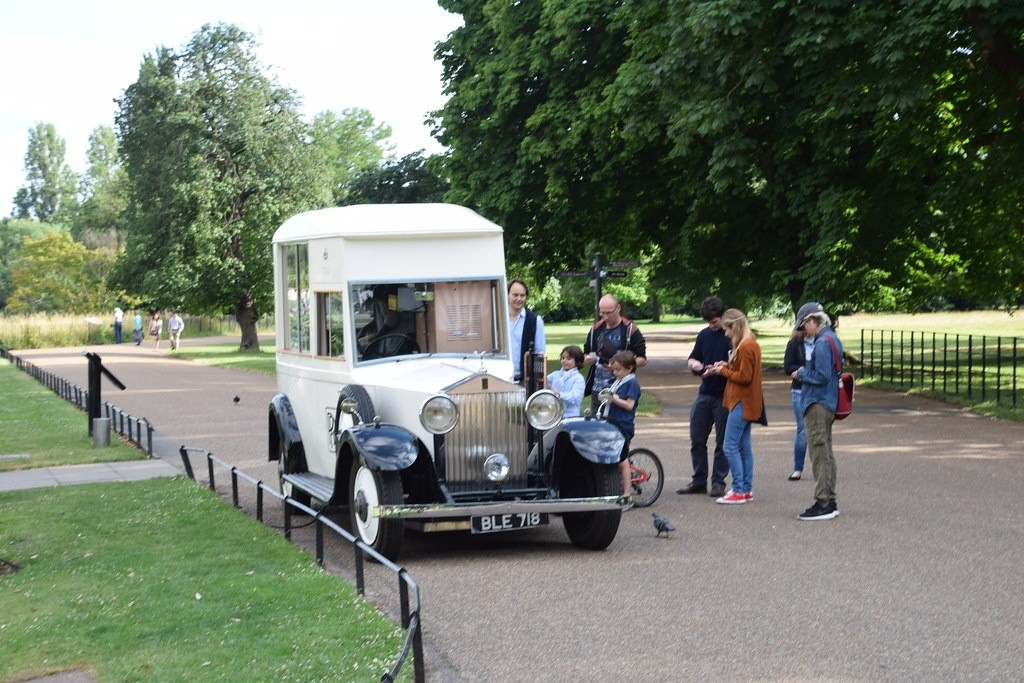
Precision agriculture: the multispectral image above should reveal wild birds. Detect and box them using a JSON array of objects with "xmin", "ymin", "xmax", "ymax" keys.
[
  {"xmin": 650, "ymin": 512, "xmax": 676, "ymax": 538},
  {"xmin": 233, "ymin": 396, "xmax": 240, "ymax": 403}
]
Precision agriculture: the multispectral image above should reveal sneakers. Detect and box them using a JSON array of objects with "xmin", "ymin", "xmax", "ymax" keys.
[
  {"xmin": 716, "ymin": 488, "xmax": 746, "ymax": 503},
  {"xmin": 675, "ymin": 482, "xmax": 708, "ymax": 494},
  {"xmin": 835, "ymin": 505, "xmax": 839, "ymax": 516},
  {"xmin": 797, "ymin": 500, "xmax": 835, "ymax": 520},
  {"xmin": 746, "ymin": 491, "xmax": 754, "ymax": 502},
  {"xmin": 710, "ymin": 487, "xmax": 725, "ymax": 497}
]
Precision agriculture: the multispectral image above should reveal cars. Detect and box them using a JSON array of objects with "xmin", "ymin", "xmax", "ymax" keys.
[{"xmin": 267, "ymin": 203, "xmax": 630, "ymax": 562}]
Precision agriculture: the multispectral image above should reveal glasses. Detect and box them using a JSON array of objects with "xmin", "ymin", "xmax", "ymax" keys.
[
  {"xmin": 800, "ymin": 320, "xmax": 808, "ymax": 329},
  {"xmin": 598, "ymin": 305, "xmax": 618, "ymax": 317}
]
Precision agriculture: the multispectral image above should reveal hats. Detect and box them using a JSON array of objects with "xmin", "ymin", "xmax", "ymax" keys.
[{"xmin": 795, "ymin": 302, "xmax": 824, "ymax": 330}]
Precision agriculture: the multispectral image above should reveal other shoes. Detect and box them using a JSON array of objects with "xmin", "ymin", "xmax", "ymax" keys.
[
  {"xmin": 788, "ymin": 472, "xmax": 802, "ymax": 481},
  {"xmin": 620, "ymin": 494, "xmax": 634, "ymax": 511}
]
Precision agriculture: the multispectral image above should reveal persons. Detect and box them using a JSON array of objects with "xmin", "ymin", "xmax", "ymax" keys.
[
  {"xmin": 147, "ymin": 313, "xmax": 162, "ymax": 349},
  {"xmin": 709, "ymin": 308, "xmax": 762, "ymax": 503},
  {"xmin": 602, "ymin": 351, "xmax": 642, "ymax": 512},
  {"xmin": 675, "ymin": 296, "xmax": 733, "ymax": 497},
  {"xmin": 507, "ymin": 279, "xmax": 545, "ymax": 390},
  {"xmin": 359, "ymin": 284, "xmax": 416, "ymax": 358},
  {"xmin": 796, "ymin": 302, "xmax": 843, "ymax": 521},
  {"xmin": 114, "ymin": 307, "xmax": 123, "ymax": 343},
  {"xmin": 583, "ymin": 294, "xmax": 647, "ymax": 417},
  {"xmin": 168, "ymin": 312, "xmax": 184, "ymax": 351},
  {"xmin": 785, "ymin": 322, "xmax": 816, "ymax": 481},
  {"xmin": 547, "ymin": 345, "xmax": 586, "ymax": 418},
  {"xmin": 134, "ymin": 310, "xmax": 142, "ymax": 345}
]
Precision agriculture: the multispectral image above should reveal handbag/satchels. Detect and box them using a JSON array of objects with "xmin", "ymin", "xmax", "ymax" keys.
[
  {"xmin": 149, "ymin": 330, "xmax": 158, "ymax": 335},
  {"xmin": 133, "ymin": 330, "xmax": 143, "ymax": 342},
  {"xmin": 835, "ymin": 373, "xmax": 856, "ymax": 420}
]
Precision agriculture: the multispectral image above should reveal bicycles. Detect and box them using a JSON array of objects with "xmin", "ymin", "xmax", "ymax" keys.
[{"xmin": 617, "ymin": 447, "xmax": 664, "ymax": 508}]
